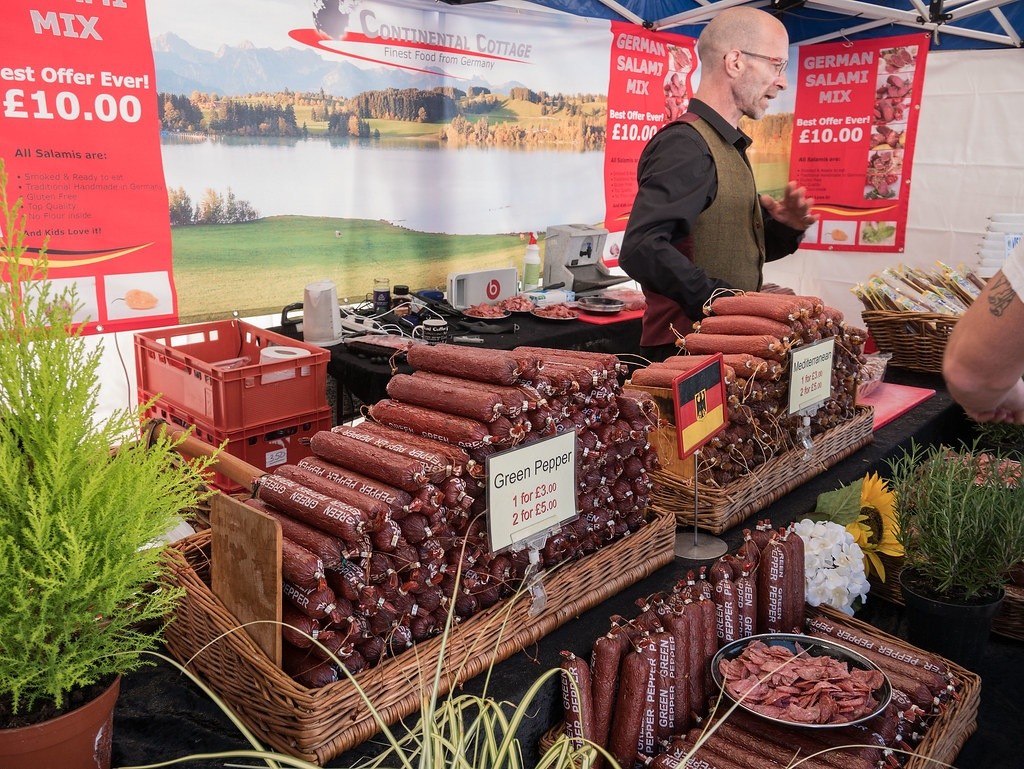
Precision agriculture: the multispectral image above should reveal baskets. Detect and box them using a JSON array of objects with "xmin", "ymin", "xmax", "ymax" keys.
[
  {"xmin": 540, "ymin": 603, "xmax": 984, "ymax": 769},
  {"xmin": 651, "ymin": 404, "xmax": 878, "ymax": 537},
  {"xmin": 862, "ymin": 450, "xmax": 1024, "ymax": 640},
  {"xmin": 157, "ymin": 503, "xmax": 671, "ymax": 764},
  {"xmin": 859, "ymin": 276, "xmax": 992, "ymax": 373}
]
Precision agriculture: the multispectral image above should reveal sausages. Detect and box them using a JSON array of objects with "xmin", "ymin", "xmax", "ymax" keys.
[
  {"xmin": 624, "ymin": 290, "xmax": 869, "ymax": 487},
  {"xmin": 243, "ymin": 334, "xmax": 664, "ymax": 690},
  {"xmin": 556, "ymin": 518, "xmax": 963, "ymax": 769},
  {"xmin": 464, "ymin": 295, "xmax": 577, "ymax": 318},
  {"xmin": 871, "ymin": 50, "xmax": 914, "ymax": 149}
]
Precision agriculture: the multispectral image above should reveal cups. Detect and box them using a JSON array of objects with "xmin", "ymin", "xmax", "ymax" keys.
[
  {"xmin": 375, "ymin": 279, "xmax": 389, "ymax": 311},
  {"xmin": 412, "ymin": 321, "xmax": 448, "ymax": 344}
]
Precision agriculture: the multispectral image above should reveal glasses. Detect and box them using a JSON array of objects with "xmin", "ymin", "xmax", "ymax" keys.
[{"xmin": 724, "ymin": 49, "xmax": 788, "ymax": 78}]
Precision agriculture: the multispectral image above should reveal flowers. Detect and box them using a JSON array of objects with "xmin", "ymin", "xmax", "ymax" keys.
[{"xmin": 791, "ymin": 467, "xmax": 908, "ymax": 616}]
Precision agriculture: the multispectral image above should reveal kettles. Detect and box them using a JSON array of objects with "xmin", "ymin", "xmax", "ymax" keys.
[{"xmin": 303, "ymin": 280, "xmax": 342, "ymax": 347}]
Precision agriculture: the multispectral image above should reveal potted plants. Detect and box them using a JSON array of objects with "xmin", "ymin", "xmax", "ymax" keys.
[
  {"xmin": 899, "ymin": 434, "xmax": 1024, "ymax": 660},
  {"xmin": 1, "ymin": 201, "xmax": 234, "ymax": 769}
]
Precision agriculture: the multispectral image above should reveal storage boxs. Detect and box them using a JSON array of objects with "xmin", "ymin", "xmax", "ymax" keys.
[{"xmin": 134, "ymin": 322, "xmax": 333, "ymax": 493}]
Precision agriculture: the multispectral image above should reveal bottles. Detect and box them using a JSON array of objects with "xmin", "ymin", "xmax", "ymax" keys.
[
  {"xmin": 390, "ymin": 284, "xmax": 412, "ymax": 316},
  {"xmin": 523, "ymin": 232, "xmax": 539, "ymax": 290}
]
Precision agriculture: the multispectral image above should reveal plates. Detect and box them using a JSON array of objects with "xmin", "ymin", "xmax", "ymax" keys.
[
  {"xmin": 711, "ymin": 633, "xmax": 891, "ymax": 729},
  {"xmin": 578, "ymin": 297, "xmax": 627, "ymax": 315},
  {"xmin": 462, "ymin": 309, "xmax": 511, "ymax": 320},
  {"xmin": 507, "ymin": 310, "xmax": 529, "ymax": 313},
  {"xmin": 530, "ymin": 308, "xmax": 580, "ymax": 320}
]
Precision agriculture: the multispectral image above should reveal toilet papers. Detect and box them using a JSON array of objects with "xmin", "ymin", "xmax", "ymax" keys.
[{"xmin": 260, "ymin": 347, "xmax": 311, "ymax": 385}]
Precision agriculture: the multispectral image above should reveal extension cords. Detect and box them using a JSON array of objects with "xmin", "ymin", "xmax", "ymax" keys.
[{"xmin": 341, "ymin": 318, "xmax": 388, "ymax": 334}]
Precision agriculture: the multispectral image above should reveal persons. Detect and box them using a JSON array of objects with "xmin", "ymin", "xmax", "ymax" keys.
[
  {"xmin": 620, "ymin": 5, "xmax": 816, "ymax": 366},
  {"xmin": 938, "ymin": 237, "xmax": 1024, "ymax": 429}
]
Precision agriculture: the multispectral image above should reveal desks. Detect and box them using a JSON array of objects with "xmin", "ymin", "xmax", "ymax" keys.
[{"xmin": 269, "ymin": 286, "xmax": 649, "ymax": 422}]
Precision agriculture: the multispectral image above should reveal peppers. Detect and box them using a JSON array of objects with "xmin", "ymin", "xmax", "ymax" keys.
[{"xmin": 111, "ymin": 289, "xmax": 158, "ymax": 309}]
